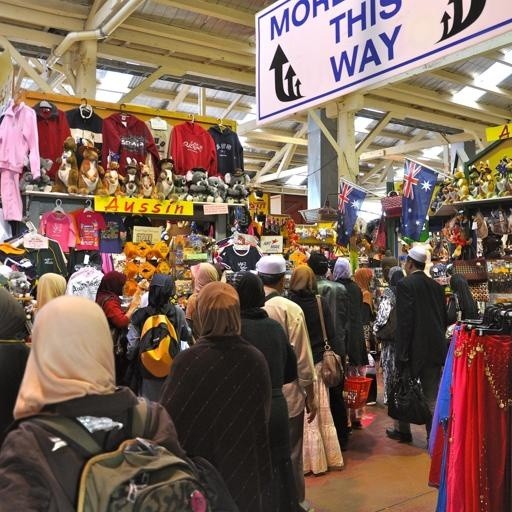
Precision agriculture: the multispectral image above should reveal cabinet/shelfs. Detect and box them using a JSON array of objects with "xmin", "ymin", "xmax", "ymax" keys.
[
  {"xmin": 443, "ymin": 139, "xmax": 511, "ymax": 305},
  {"xmin": 241, "ymin": 190, "xmax": 335, "ymax": 254},
  {"xmin": 15, "ymin": 87, "xmax": 246, "ymax": 242}
]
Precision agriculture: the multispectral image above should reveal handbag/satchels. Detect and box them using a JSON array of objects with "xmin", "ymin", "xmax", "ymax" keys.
[
  {"xmin": 449, "ymin": 240, "xmax": 489, "ymax": 282},
  {"xmin": 468, "ymin": 279, "xmax": 490, "ymax": 302},
  {"xmin": 380, "ymin": 189, "xmax": 403, "ymax": 212}
]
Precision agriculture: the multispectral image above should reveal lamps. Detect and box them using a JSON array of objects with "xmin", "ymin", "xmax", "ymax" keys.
[{"xmin": 253, "ymin": 188, "xmax": 264, "ymax": 199}]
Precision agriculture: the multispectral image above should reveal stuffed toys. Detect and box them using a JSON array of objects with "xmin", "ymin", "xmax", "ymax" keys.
[
  {"xmin": 103, "ymin": 158, "xmax": 125, "ymax": 195},
  {"xmin": 224, "ymin": 168, "xmax": 250, "ymax": 204},
  {"xmin": 453, "ymin": 168, "xmax": 470, "ymax": 200},
  {"xmin": 207, "ymin": 175, "xmax": 227, "ymax": 203},
  {"xmin": 121, "ymin": 156, "xmax": 139, "ymax": 196},
  {"xmin": 186, "ymin": 167, "xmax": 210, "ymax": 201},
  {"xmin": 476, "ymin": 159, "xmax": 495, "ymax": 198},
  {"xmin": 467, "ymin": 164, "xmax": 479, "ymax": 199},
  {"xmin": 431, "ymin": 182, "xmax": 445, "ymax": 212},
  {"xmin": 17, "ymin": 152, "xmax": 54, "ymax": 194},
  {"xmin": 52, "ymin": 136, "xmax": 79, "ymax": 194},
  {"xmin": 176, "ymin": 174, "xmax": 194, "ymax": 201},
  {"xmin": 79, "ymin": 143, "xmax": 105, "ymax": 194},
  {"xmin": 157, "ymin": 157, "xmax": 181, "ymax": 200},
  {"xmin": 139, "ymin": 161, "xmax": 156, "ymax": 198}
]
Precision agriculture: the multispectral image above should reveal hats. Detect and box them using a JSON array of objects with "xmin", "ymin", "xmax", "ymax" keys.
[
  {"xmin": 408, "ymin": 246, "xmax": 428, "ymax": 264},
  {"xmin": 255, "ymin": 255, "xmax": 286, "ymax": 275}
]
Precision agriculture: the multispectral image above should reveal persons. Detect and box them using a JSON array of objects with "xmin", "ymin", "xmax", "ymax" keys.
[
  {"xmin": 0, "ymin": 294, "xmax": 200, "ymax": 512},
  {"xmin": 493, "ymin": 157, "xmax": 508, "ymax": 197},
  {"xmin": 441, "ymin": 175, "xmax": 456, "ymax": 203},
  {"xmin": 445, "ymin": 270, "xmax": 480, "ymax": 346},
  {"xmin": 352, "ymin": 266, "xmax": 375, "ymax": 425},
  {"xmin": 371, "ymin": 265, "xmax": 406, "ymax": 406},
  {"xmin": 254, "ymin": 252, "xmax": 318, "ymax": 512},
  {"xmin": 0, "ymin": 283, "xmax": 35, "ymax": 441},
  {"xmin": 157, "ymin": 280, "xmax": 275, "ymax": 512},
  {"xmin": 94, "ymin": 270, "xmax": 143, "ymax": 364},
  {"xmin": 183, "ymin": 262, "xmax": 220, "ymax": 329},
  {"xmin": 225, "ymin": 269, "xmax": 299, "ymax": 512},
  {"xmin": 285, "ymin": 263, "xmax": 345, "ymax": 477},
  {"xmin": 383, "ymin": 246, "xmax": 448, "ymax": 450},
  {"xmin": 326, "ymin": 255, "xmax": 371, "ymax": 429},
  {"xmin": 34, "ymin": 271, "xmax": 67, "ymax": 313},
  {"xmin": 307, "ymin": 250, "xmax": 350, "ymax": 452},
  {"xmin": 500, "ymin": 158, "xmax": 512, "ymax": 196},
  {"xmin": 428, "ymin": 262, "xmax": 453, "ymax": 303}
]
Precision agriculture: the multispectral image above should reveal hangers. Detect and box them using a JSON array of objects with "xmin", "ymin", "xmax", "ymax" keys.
[
  {"xmin": 463, "ymin": 307, "xmax": 507, "ymax": 332},
  {"xmin": 486, "ymin": 302, "xmax": 512, "ymax": 317},
  {"xmin": 153, "ymin": 109, "xmax": 163, "ymax": 120},
  {"xmin": 12, "ymin": 88, "xmax": 27, "ymax": 113},
  {"xmin": 188, "ymin": 114, "xmax": 194, "ymax": 124},
  {"xmin": 474, "ymin": 309, "xmax": 512, "ymax": 336},
  {"xmin": 457, "ymin": 307, "xmax": 496, "ymax": 324},
  {"xmin": 51, "ymin": 199, "xmax": 67, "ymax": 216},
  {"xmin": 119, "ymin": 104, "xmax": 132, "ymax": 117},
  {"xmin": 465, "ymin": 304, "xmax": 495, "ymax": 322},
  {"xmin": 217, "ymin": 117, "xmax": 227, "ymax": 128},
  {"xmin": 81, "ymin": 98, "xmax": 90, "ymax": 113},
  {"xmin": 40, "ymin": 92, "xmax": 53, "ymax": 109},
  {"xmin": 132, "ymin": 213, "xmax": 143, "ymax": 217},
  {"xmin": 83, "ymin": 199, "xmax": 95, "ymax": 213}
]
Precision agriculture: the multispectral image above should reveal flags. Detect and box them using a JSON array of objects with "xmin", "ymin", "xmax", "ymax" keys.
[
  {"xmin": 398, "ymin": 156, "xmax": 441, "ymax": 243},
  {"xmin": 334, "ymin": 176, "xmax": 371, "ymax": 248}
]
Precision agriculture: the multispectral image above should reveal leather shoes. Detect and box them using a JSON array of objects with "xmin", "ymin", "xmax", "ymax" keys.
[{"xmin": 386, "ymin": 426, "xmax": 412, "ymax": 444}]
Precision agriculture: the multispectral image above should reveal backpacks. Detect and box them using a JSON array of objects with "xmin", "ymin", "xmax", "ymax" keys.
[
  {"xmin": 138, "ymin": 305, "xmax": 183, "ymax": 378},
  {"xmin": 10, "ymin": 396, "xmax": 239, "ymax": 512}
]
